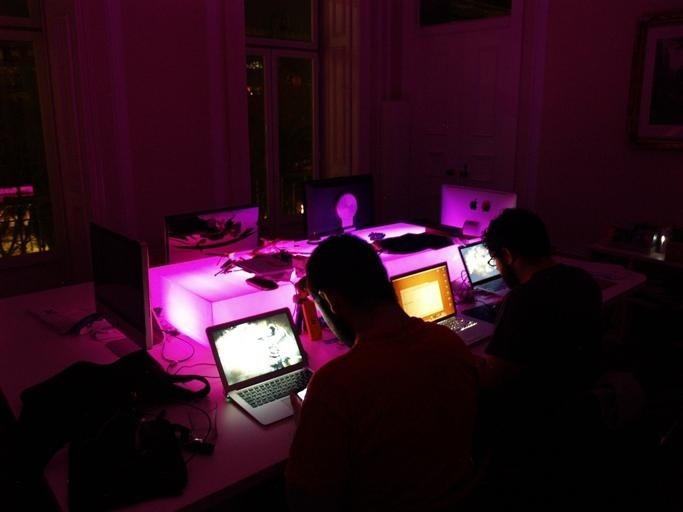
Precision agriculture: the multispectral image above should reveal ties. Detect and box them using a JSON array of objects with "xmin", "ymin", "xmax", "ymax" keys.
[
  {"xmin": 303, "ymin": 174, "xmax": 375, "ymax": 240},
  {"xmin": 67, "ymin": 223, "xmax": 164, "ymax": 359},
  {"xmin": 439, "ymin": 183, "xmax": 518, "ymax": 240}
]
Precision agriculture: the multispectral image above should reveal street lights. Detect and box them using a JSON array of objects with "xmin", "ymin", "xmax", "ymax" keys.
[{"xmin": 20, "ymin": 350, "xmax": 211, "ymax": 426}]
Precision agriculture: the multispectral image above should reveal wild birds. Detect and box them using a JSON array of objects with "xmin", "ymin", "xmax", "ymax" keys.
[
  {"xmin": 458, "ymin": 241, "xmax": 512, "ymax": 297},
  {"xmin": 206, "ymin": 307, "xmax": 315, "ymax": 426},
  {"xmin": 390, "ymin": 261, "xmax": 497, "ymax": 347}
]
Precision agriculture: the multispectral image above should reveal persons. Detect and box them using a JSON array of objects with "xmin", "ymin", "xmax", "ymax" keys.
[
  {"xmin": 282, "ymin": 235, "xmax": 475, "ymax": 511},
  {"xmin": 469, "ymin": 207, "xmax": 617, "ymax": 512}
]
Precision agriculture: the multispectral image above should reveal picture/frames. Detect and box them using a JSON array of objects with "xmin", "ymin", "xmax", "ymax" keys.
[{"xmin": 0, "ymin": 222, "xmax": 646, "ymax": 512}]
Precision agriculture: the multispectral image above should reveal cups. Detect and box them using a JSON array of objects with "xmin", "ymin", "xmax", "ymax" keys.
[{"xmin": 487, "ymin": 255, "xmax": 498, "ymax": 266}]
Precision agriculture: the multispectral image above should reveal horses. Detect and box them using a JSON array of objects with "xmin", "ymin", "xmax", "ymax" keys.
[{"xmin": 295, "ymin": 385, "xmax": 308, "ymax": 401}]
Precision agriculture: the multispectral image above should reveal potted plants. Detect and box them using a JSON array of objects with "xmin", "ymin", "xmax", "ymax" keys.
[
  {"xmin": 415, "ymin": 0, "xmax": 512, "ymax": 27},
  {"xmin": 630, "ymin": 7, "xmax": 682, "ymax": 155}
]
